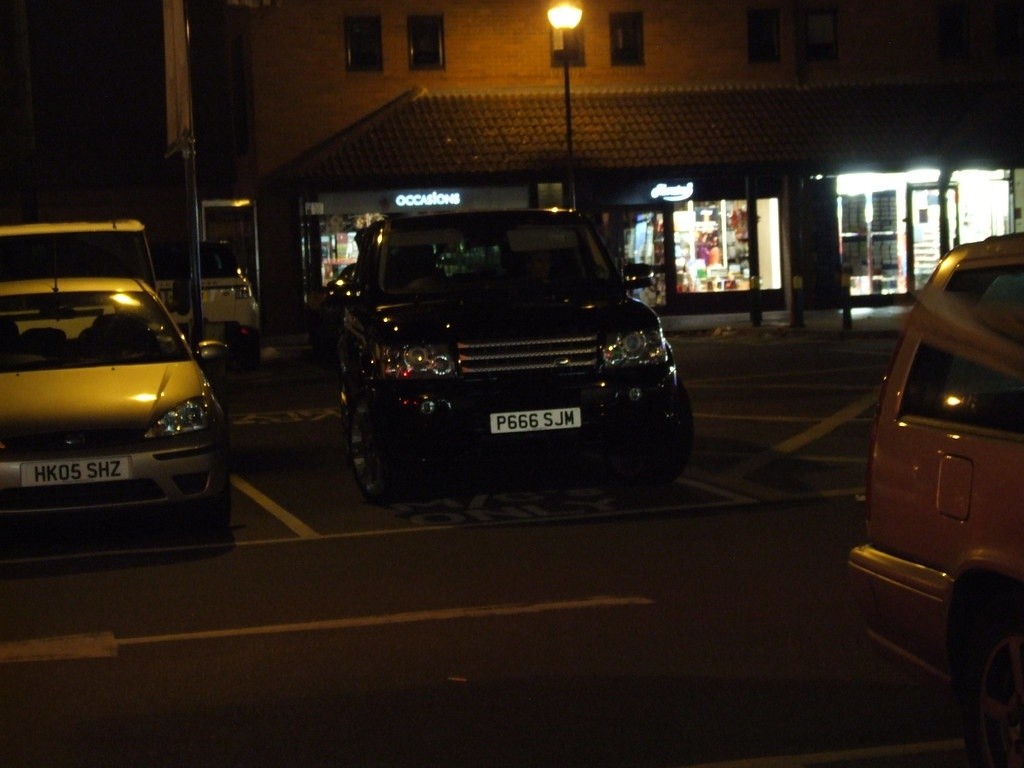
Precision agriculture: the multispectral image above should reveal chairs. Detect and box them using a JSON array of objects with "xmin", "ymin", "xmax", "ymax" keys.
[{"xmin": 90, "ymin": 315, "xmax": 159, "ymax": 357}]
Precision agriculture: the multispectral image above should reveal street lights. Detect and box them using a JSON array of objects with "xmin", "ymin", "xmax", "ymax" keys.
[{"xmin": 546, "ymin": 0, "xmax": 585, "ymax": 210}]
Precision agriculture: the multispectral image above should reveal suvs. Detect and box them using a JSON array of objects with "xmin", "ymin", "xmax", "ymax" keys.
[
  {"xmin": 843, "ymin": 231, "xmax": 1023, "ymax": 768},
  {"xmin": 0, "ymin": 222, "xmax": 191, "ymax": 339},
  {"xmin": 150, "ymin": 239, "xmax": 260, "ymax": 371},
  {"xmin": 326, "ymin": 206, "xmax": 695, "ymax": 506}
]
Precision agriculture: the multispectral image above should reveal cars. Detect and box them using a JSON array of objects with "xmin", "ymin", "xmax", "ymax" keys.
[
  {"xmin": 306, "ymin": 264, "xmax": 357, "ymax": 364},
  {"xmin": 0, "ymin": 277, "xmax": 233, "ymax": 541}
]
